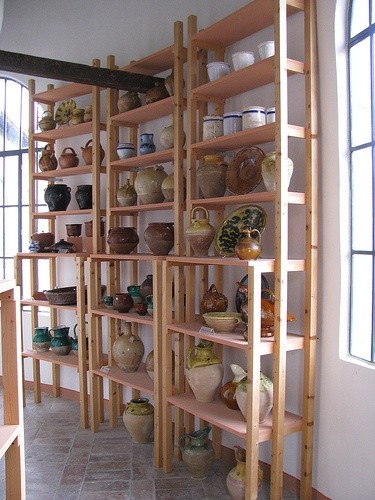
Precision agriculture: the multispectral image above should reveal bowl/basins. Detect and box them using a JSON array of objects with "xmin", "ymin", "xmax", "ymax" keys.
[
  {"xmin": 203, "ymin": 313, "xmax": 241, "ymax": 334},
  {"xmin": 66, "ymin": 224, "xmax": 82, "ymax": 237},
  {"xmin": 44, "ymin": 285, "xmax": 106, "ymax": 305}
]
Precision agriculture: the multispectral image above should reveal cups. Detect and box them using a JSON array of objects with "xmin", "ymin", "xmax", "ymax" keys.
[
  {"xmin": 233, "ymin": 52, "xmax": 254, "ymax": 71},
  {"xmin": 242, "ymin": 106, "xmax": 266, "ymax": 131},
  {"xmin": 258, "ymin": 41, "xmax": 275, "ymax": 60},
  {"xmin": 207, "ymin": 62, "xmax": 231, "ymax": 81},
  {"xmin": 203, "ymin": 116, "xmax": 223, "ymax": 141},
  {"xmin": 266, "ymin": 107, "xmax": 275, "ymax": 124},
  {"xmin": 224, "ymin": 112, "xmax": 242, "ymax": 137}
]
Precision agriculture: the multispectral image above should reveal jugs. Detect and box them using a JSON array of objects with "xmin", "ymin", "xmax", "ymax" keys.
[
  {"xmin": 235, "ymin": 227, "xmax": 261, "ymax": 259},
  {"xmin": 226, "ymin": 445, "xmax": 263, "ymax": 500},
  {"xmin": 49, "ymin": 326, "xmax": 72, "ymax": 355},
  {"xmin": 186, "ymin": 207, "xmax": 214, "ymax": 257},
  {"xmin": 185, "ymin": 340, "xmax": 224, "ymax": 403},
  {"xmin": 179, "ymin": 426, "xmax": 215, "ymax": 481}
]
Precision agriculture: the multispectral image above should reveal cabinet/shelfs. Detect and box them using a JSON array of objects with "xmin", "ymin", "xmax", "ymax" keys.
[{"xmin": 17, "ymin": 0, "xmax": 318, "ymax": 500}]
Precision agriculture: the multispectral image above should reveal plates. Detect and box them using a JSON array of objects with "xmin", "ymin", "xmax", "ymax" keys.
[
  {"xmin": 215, "ymin": 205, "xmax": 267, "ymax": 257},
  {"xmin": 225, "ymin": 146, "xmax": 266, "ymax": 195},
  {"xmin": 56, "ymin": 100, "xmax": 75, "ymax": 126},
  {"xmin": 235, "ymin": 274, "xmax": 269, "ymax": 321}
]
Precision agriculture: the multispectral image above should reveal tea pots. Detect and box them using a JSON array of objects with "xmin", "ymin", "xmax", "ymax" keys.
[
  {"xmin": 59, "ymin": 147, "xmax": 78, "ymax": 168},
  {"xmin": 40, "ymin": 111, "xmax": 55, "ymax": 130},
  {"xmin": 39, "ymin": 144, "xmax": 57, "ymax": 171},
  {"xmin": 81, "ymin": 139, "xmax": 104, "ymax": 164}
]
